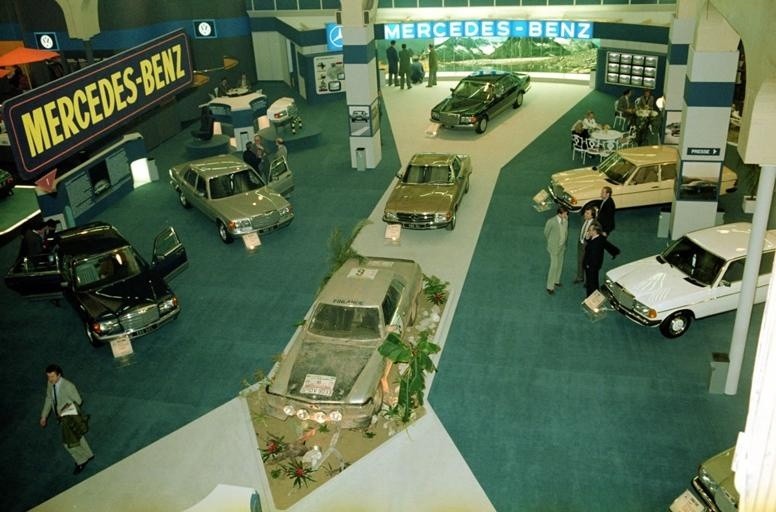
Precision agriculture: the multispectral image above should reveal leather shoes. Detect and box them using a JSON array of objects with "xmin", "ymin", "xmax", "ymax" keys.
[
  {"xmin": 544, "ymin": 281, "xmax": 562, "ymax": 295},
  {"xmin": 574, "ymin": 278, "xmax": 587, "ymax": 292},
  {"xmin": 72, "ymin": 455, "xmax": 95, "ymax": 475},
  {"xmin": 610, "ymin": 250, "xmax": 624, "ymax": 262}
]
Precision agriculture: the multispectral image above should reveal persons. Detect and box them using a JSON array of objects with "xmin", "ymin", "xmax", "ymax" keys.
[
  {"xmin": 218, "ymin": 78, "xmax": 231, "ymax": 97},
  {"xmin": 236, "ymin": 73, "xmax": 252, "ymax": 90},
  {"xmin": 386, "ymin": 40, "xmax": 439, "ymax": 90},
  {"xmin": 244, "ymin": 142, "xmax": 265, "ymax": 175},
  {"xmin": 543, "ymin": 186, "xmax": 621, "ymax": 296},
  {"xmin": 274, "ymin": 139, "xmax": 289, "ymax": 173},
  {"xmin": 254, "ymin": 135, "xmax": 266, "ymax": 162},
  {"xmin": 15, "ymin": 222, "xmax": 49, "ymax": 305},
  {"xmin": 572, "ymin": 88, "xmax": 654, "ymax": 158},
  {"xmin": 38, "ymin": 365, "xmax": 93, "ymax": 473}
]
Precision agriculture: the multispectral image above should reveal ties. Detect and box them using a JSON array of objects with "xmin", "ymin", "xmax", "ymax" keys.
[
  {"xmin": 581, "ymin": 221, "xmax": 588, "ymax": 240},
  {"xmin": 51, "ymin": 384, "xmax": 60, "ymax": 421}
]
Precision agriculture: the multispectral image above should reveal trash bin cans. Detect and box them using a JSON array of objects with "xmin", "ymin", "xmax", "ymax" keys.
[
  {"xmin": 715, "ymin": 208, "xmax": 727, "ymax": 227},
  {"xmin": 656, "ymin": 206, "xmax": 672, "ymax": 239},
  {"xmin": 355, "ymin": 147, "xmax": 367, "ymax": 173},
  {"xmin": 708, "ymin": 352, "xmax": 731, "ymax": 394},
  {"xmin": 146, "ymin": 158, "xmax": 161, "ymax": 182}
]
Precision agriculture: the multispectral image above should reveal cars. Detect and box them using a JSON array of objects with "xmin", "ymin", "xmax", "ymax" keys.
[
  {"xmin": 431, "ymin": 71, "xmax": 533, "ymax": 133},
  {"xmin": 168, "ymin": 152, "xmax": 298, "ymax": 246},
  {"xmin": 599, "ymin": 221, "xmax": 776, "ymax": 338},
  {"xmin": 0, "ymin": 170, "xmax": 14, "ymax": 199},
  {"xmin": 265, "ymin": 253, "xmax": 421, "ymax": 432},
  {"xmin": 548, "ymin": 144, "xmax": 738, "ymax": 216},
  {"xmin": 381, "ymin": 152, "xmax": 472, "ymax": 232},
  {"xmin": 4, "ymin": 221, "xmax": 188, "ymax": 349}
]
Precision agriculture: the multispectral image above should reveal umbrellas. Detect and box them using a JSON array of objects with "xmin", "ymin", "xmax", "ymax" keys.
[{"xmin": 0, "ymin": 45, "xmax": 59, "ymax": 90}]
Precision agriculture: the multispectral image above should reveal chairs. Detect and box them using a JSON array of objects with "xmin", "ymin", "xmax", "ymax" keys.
[
  {"xmin": 75, "ymin": 263, "xmax": 101, "ymax": 288},
  {"xmin": 185, "ymin": 88, "xmax": 279, "ymax": 162},
  {"xmin": 569, "ymin": 95, "xmax": 664, "ymax": 165},
  {"xmin": 431, "ymin": 166, "xmax": 450, "ymax": 185},
  {"xmin": 408, "ymin": 164, "xmax": 424, "ymax": 182}
]
